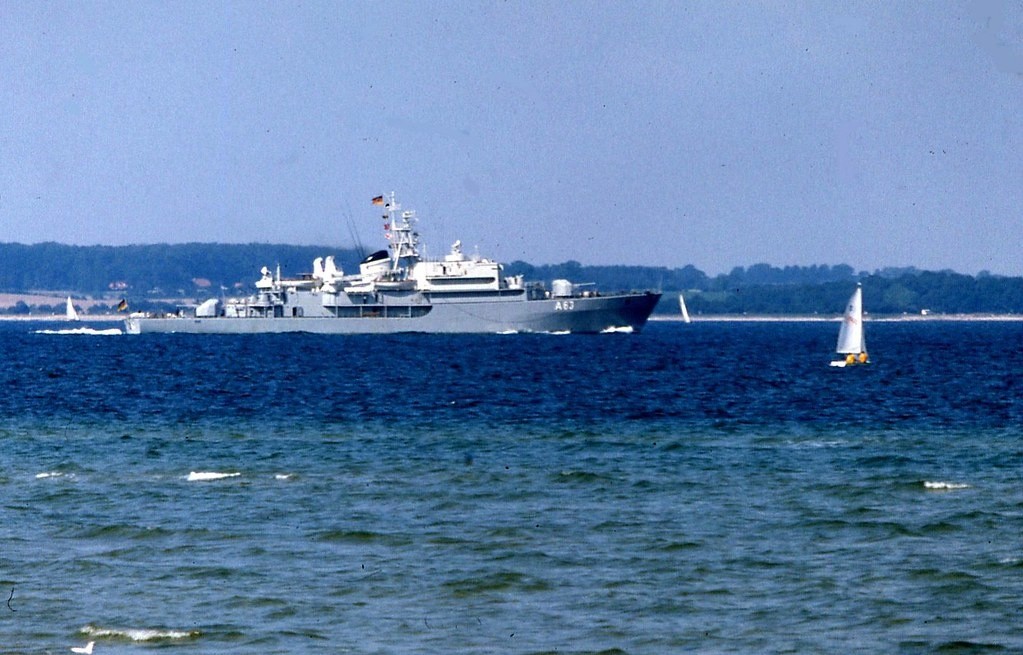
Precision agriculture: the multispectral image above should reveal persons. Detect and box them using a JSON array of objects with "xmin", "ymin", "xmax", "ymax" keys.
[{"xmin": 845, "ymin": 351, "xmax": 867, "ymax": 364}]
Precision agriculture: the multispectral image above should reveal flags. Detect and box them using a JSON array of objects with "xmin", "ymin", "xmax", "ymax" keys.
[
  {"xmin": 372, "ymin": 195, "xmax": 382, "ymax": 205},
  {"xmin": 117, "ymin": 299, "xmax": 127, "ymax": 312}
]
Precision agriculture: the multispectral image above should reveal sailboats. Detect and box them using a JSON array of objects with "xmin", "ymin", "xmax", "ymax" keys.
[
  {"xmin": 680, "ymin": 295, "xmax": 692, "ymax": 324},
  {"xmin": 828, "ymin": 282, "xmax": 872, "ymax": 368},
  {"xmin": 66, "ymin": 296, "xmax": 80, "ymax": 322}
]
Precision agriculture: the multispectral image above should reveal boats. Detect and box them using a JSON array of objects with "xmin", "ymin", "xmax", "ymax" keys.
[
  {"xmin": 374, "ymin": 279, "xmax": 418, "ymax": 292},
  {"xmin": 121, "ymin": 190, "xmax": 665, "ymax": 336}
]
